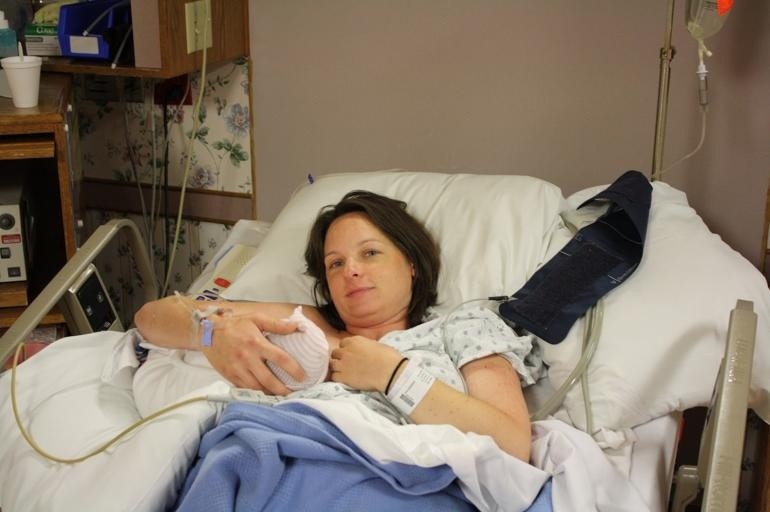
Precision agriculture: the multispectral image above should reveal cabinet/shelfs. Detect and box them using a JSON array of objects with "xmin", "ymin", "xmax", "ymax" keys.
[
  {"xmin": 40, "ymin": 1, "xmax": 249, "ymax": 79},
  {"xmin": 1, "ymin": 77, "xmax": 80, "ymax": 328}
]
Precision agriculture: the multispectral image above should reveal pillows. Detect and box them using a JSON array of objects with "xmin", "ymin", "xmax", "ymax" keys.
[{"xmin": 539, "ymin": 182, "xmax": 770, "ymax": 437}]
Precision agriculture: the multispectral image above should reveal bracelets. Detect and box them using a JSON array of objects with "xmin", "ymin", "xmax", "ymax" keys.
[{"xmin": 381, "ymin": 356, "xmax": 437, "ymax": 419}]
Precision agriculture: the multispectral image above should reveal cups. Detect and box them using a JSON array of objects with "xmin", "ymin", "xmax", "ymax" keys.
[{"xmin": 0, "ymin": 55, "xmax": 43, "ymax": 108}]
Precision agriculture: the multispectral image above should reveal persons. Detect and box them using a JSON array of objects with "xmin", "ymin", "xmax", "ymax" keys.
[{"xmin": 132, "ymin": 189, "xmax": 547, "ymax": 471}]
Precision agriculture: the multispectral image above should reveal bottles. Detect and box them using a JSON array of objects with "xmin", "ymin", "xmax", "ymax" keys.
[{"xmin": 0, "ymin": 10, "xmax": 19, "ymax": 99}]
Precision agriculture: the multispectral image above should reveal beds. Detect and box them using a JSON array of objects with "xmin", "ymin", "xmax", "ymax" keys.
[{"xmin": 0, "ymin": 217, "xmax": 765, "ymax": 510}]
